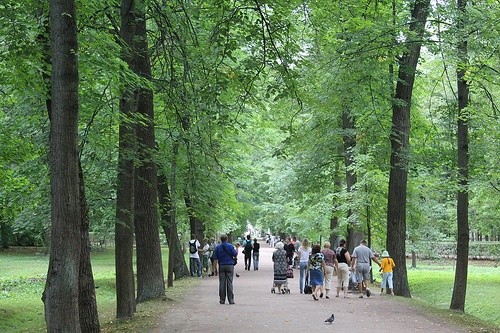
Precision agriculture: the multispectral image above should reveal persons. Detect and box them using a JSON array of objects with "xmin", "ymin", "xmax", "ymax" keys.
[
  {"xmin": 335, "ymin": 239, "xmax": 352, "ymax": 298},
  {"xmin": 379, "ymin": 251, "xmax": 397, "ymax": 295},
  {"xmin": 352, "ymin": 240, "xmax": 381, "ymax": 298},
  {"xmin": 202, "ymin": 238, "xmax": 209, "ymax": 274},
  {"xmin": 189, "ymin": 234, "xmax": 204, "ymax": 278},
  {"xmin": 320, "ymin": 242, "xmax": 338, "ymax": 299},
  {"xmin": 292, "ymin": 235, "xmax": 301, "ymax": 269},
  {"xmin": 298, "ymin": 239, "xmax": 312, "ymax": 294},
  {"xmin": 208, "ymin": 237, "xmax": 217, "ymax": 276},
  {"xmin": 252, "ymin": 238, "xmax": 261, "ymax": 270},
  {"xmin": 272, "ymin": 242, "xmax": 288, "ymax": 294},
  {"xmin": 243, "ymin": 235, "xmax": 254, "ymax": 271},
  {"xmin": 285, "ymin": 237, "xmax": 295, "ymax": 278},
  {"xmin": 308, "ymin": 245, "xmax": 328, "ymax": 301},
  {"xmin": 212, "ymin": 234, "xmax": 241, "ymax": 304}
]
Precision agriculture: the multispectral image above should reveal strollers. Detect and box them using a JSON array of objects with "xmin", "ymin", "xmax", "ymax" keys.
[{"xmin": 271, "ymin": 281, "xmax": 292, "ymax": 294}]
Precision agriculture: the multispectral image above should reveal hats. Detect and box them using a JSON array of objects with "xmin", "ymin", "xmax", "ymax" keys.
[{"xmin": 382, "ymin": 251, "xmax": 389, "ymax": 257}]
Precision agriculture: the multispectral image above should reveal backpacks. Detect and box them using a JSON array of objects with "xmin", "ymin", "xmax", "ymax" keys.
[
  {"xmin": 189, "ymin": 239, "xmax": 197, "ymax": 254},
  {"xmin": 245, "ymin": 240, "xmax": 252, "ymax": 252}
]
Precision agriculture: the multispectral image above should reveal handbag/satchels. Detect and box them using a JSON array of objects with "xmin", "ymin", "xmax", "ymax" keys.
[
  {"xmin": 304, "ymin": 275, "xmax": 312, "ymax": 294},
  {"xmin": 233, "ymin": 258, "xmax": 237, "ymax": 266}
]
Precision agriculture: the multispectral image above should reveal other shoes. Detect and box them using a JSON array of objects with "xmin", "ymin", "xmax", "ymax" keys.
[
  {"xmin": 359, "ymin": 296, "xmax": 363, "ymax": 298},
  {"xmin": 312, "ymin": 294, "xmax": 318, "ymax": 300},
  {"xmin": 326, "ymin": 296, "xmax": 329, "ymax": 298},
  {"xmin": 366, "ymin": 289, "xmax": 370, "ymax": 297},
  {"xmin": 320, "ymin": 293, "xmax": 323, "ymax": 297}
]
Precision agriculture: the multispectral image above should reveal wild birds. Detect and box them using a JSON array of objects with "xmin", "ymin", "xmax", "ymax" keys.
[
  {"xmin": 325, "ymin": 313, "xmax": 334, "ymax": 323},
  {"xmin": 236, "ymin": 273, "xmax": 239, "ymax": 277}
]
